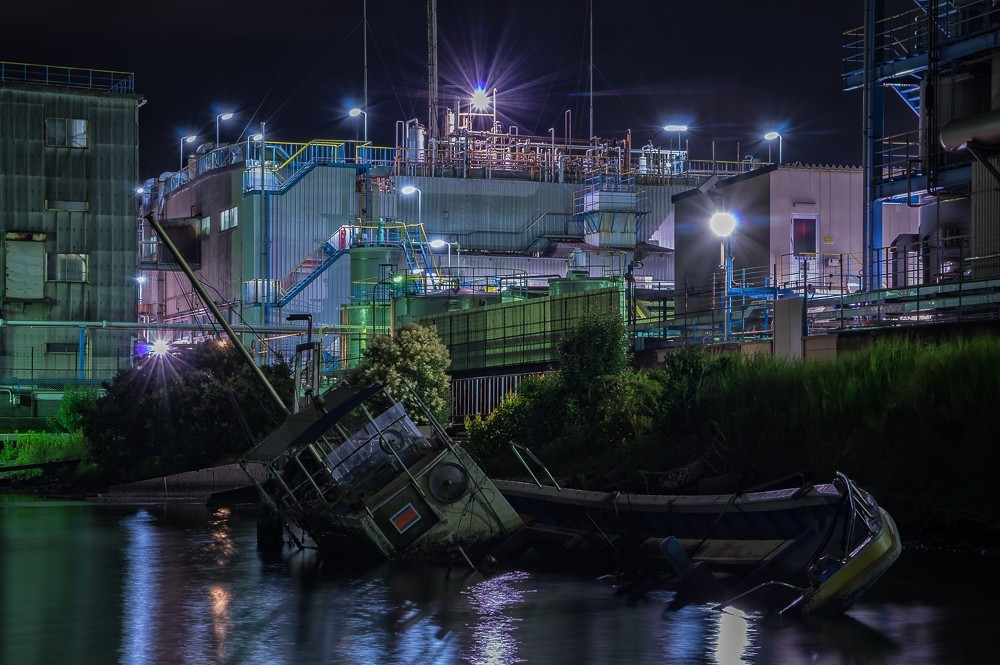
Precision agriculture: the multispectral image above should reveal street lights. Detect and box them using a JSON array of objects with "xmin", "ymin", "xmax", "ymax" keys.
[
  {"xmin": 664, "ymin": 125, "xmax": 689, "ymax": 174},
  {"xmin": 765, "ymin": 132, "xmax": 782, "ymax": 163},
  {"xmin": 431, "ymin": 240, "xmax": 450, "ymax": 268},
  {"xmin": 349, "ymin": 109, "xmax": 367, "ymax": 145},
  {"xmin": 710, "ymin": 211, "xmax": 737, "ymax": 341},
  {"xmin": 181, "ymin": 135, "xmax": 197, "ymax": 170},
  {"xmin": 402, "ymin": 186, "xmax": 421, "ymax": 224},
  {"xmin": 217, "ymin": 112, "xmax": 235, "ymax": 149}
]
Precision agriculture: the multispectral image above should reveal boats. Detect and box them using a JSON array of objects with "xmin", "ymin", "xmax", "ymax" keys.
[{"xmin": 142, "ymin": 210, "xmax": 903, "ymax": 630}]
